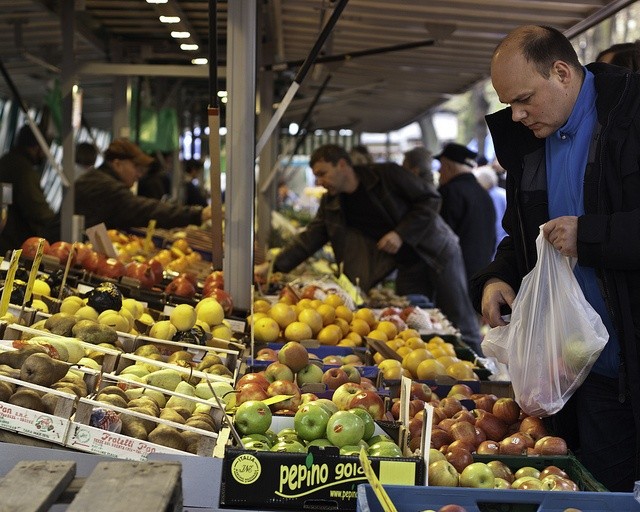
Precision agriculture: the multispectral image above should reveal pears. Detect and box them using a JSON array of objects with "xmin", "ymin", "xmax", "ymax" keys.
[
  {"xmin": 26, "ymin": 333, "xmax": 236, "ymax": 411},
  {"xmin": 0, "ymin": 340, "xmax": 219, "ymax": 461},
  {"xmin": 33, "ymin": 313, "xmax": 233, "ymax": 384}
]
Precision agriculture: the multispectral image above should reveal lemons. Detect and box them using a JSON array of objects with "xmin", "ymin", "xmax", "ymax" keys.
[{"xmin": 235, "ymin": 293, "xmax": 482, "ymax": 385}]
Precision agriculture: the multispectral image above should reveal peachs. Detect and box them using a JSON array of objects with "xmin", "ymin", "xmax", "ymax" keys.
[{"xmin": 40, "ymin": 292, "xmax": 235, "ymax": 352}]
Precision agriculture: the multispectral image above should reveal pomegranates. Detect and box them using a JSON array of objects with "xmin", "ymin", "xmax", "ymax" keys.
[{"xmin": 15, "ymin": 236, "xmax": 234, "ymax": 319}]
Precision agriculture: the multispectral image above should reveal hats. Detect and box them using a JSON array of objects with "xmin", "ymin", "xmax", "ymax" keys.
[
  {"xmin": 403, "ymin": 146, "xmax": 431, "ymax": 170},
  {"xmin": 432, "ymin": 143, "xmax": 478, "ymax": 166},
  {"xmin": 103, "ymin": 137, "xmax": 154, "ymax": 170}
]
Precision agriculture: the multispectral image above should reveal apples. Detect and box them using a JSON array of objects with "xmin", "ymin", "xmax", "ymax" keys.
[
  {"xmin": 412, "ymin": 444, "xmax": 580, "ymax": 492},
  {"xmin": 389, "ymin": 381, "xmax": 569, "ymax": 455},
  {"xmin": 231, "ymin": 397, "xmax": 403, "ymax": 459},
  {"xmin": 235, "ymin": 340, "xmax": 383, "ymax": 407}
]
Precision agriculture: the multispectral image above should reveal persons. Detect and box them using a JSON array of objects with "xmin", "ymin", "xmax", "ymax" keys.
[
  {"xmin": 74, "ymin": 142, "xmax": 97, "ymax": 181},
  {"xmin": 56, "ymin": 138, "xmax": 226, "ymax": 230},
  {"xmin": 435, "ymin": 142, "xmax": 496, "ymax": 283},
  {"xmin": 402, "ymin": 148, "xmax": 433, "ymax": 187},
  {"xmin": 468, "ymin": 166, "xmax": 508, "ymax": 260},
  {"xmin": 160, "ymin": 151, "xmax": 176, "ymax": 198},
  {"xmin": 350, "ymin": 146, "xmax": 373, "ymax": 165},
  {"xmin": 178, "ymin": 159, "xmax": 208, "ymax": 207},
  {"xmin": 273, "ymin": 144, "xmax": 480, "ymax": 340},
  {"xmin": 468, "ymin": 24, "xmax": 640, "ymax": 492},
  {"xmin": 0, "ymin": 123, "xmax": 89, "ymax": 253},
  {"xmin": 138, "ymin": 150, "xmax": 174, "ymax": 199}
]
